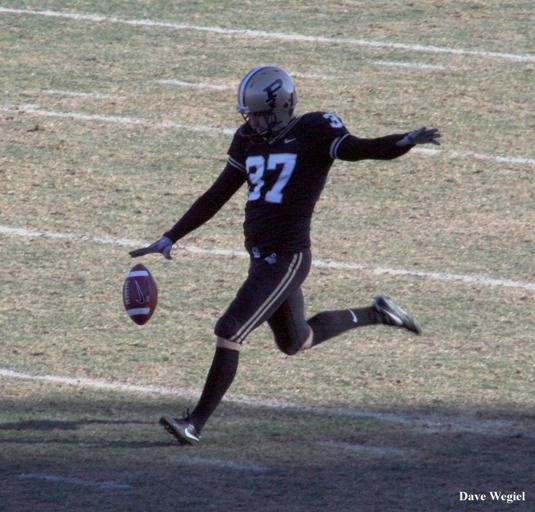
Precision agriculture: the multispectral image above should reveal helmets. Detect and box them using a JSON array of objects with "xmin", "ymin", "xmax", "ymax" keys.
[{"xmin": 236, "ymin": 65, "xmax": 298, "ymax": 137}]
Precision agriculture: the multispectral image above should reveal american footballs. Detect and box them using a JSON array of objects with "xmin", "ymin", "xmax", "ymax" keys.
[{"xmin": 123, "ymin": 264, "xmax": 157, "ymax": 325}]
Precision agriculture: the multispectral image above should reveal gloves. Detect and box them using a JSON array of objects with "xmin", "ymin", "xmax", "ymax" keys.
[
  {"xmin": 396, "ymin": 126, "xmax": 443, "ymax": 146},
  {"xmin": 129, "ymin": 235, "xmax": 174, "ymax": 261}
]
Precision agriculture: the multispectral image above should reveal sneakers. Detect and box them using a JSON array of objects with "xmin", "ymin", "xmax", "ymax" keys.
[
  {"xmin": 160, "ymin": 414, "xmax": 200, "ymax": 445},
  {"xmin": 373, "ymin": 295, "xmax": 422, "ymax": 337}
]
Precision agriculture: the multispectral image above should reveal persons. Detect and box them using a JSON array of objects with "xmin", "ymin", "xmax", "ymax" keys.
[{"xmin": 128, "ymin": 66, "xmax": 443, "ymax": 446}]
